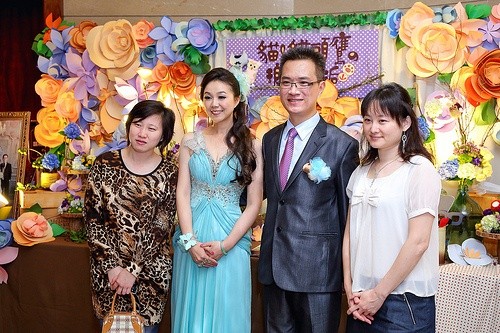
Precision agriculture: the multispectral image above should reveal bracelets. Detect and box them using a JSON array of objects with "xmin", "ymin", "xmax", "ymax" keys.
[
  {"xmin": 177, "ymin": 233, "xmax": 199, "ymax": 251},
  {"xmin": 220, "ymin": 240, "xmax": 228, "ymax": 256}
]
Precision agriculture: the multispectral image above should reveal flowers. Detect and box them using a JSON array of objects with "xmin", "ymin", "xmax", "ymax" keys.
[
  {"xmin": 251, "ymin": 81, "xmax": 371, "ymax": 242},
  {"xmin": 384, "ymin": 1, "xmax": 500, "ymax": 267},
  {"xmin": 0, "ymin": 14, "xmax": 219, "ymax": 283}
]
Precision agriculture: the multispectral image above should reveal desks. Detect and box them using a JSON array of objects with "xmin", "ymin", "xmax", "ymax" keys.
[{"xmin": 0, "ymin": 236, "xmax": 347, "ymax": 333}]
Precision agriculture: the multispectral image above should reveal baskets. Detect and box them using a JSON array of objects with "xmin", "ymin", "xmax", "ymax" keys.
[{"xmin": 57, "ymin": 213, "xmax": 83, "ymax": 237}]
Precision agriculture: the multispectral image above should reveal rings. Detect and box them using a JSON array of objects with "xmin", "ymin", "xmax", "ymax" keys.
[{"xmin": 198, "ymin": 259, "xmax": 210, "ymax": 267}]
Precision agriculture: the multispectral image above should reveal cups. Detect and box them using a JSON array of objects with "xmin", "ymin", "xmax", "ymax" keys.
[{"xmin": 0, "ymin": 206, "xmax": 12, "ymax": 221}]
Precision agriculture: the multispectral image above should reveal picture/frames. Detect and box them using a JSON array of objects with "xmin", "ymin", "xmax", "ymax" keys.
[{"xmin": 0, "ymin": 111, "xmax": 31, "ymax": 220}]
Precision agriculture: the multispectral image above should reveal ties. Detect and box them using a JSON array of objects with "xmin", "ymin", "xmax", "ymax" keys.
[{"xmin": 279, "ymin": 128, "xmax": 298, "ymax": 193}]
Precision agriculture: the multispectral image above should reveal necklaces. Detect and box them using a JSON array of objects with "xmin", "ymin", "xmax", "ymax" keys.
[{"xmin": 373, "ymin": 154, "xmax": 400, "ymax": 179}]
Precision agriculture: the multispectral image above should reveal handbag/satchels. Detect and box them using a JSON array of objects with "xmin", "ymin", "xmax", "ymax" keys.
[{"xmin": 102, "ymin": 290, "xmax": 144, "ymax": 333}]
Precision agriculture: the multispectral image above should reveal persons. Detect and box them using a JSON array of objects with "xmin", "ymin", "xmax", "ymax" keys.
[
  {"xmin": 342, "ymin": 82, "xmax": 442, "ymax": 333},
  {"xmin": 83, "ymin": 100, "xmax": 179, "ymax": 333},
  {"xmin": 0, "ymin": 154, "xmax": 12, "ymax": 196},
  {"xmin": 240, "ymin": 48, "xmax": 359, "ymax": 333},
  {"xmin": 171, "ymin": 67, "xmax": 264, "ymax": 333}
]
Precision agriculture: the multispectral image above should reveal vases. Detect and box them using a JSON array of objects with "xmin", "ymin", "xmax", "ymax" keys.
[
  {"xmin": 475, "ymin": 230, "xmax": 500, "ymax": 264},
  {"xmin": 41, "ymin": 171, "xmax": 59, "ymax": 189},
  {"xmin": 445, "ymin": 185, "xmax": 485, "ymax": 261},
  {"xmin": 60, "ymin": 213, "xmax": 84, "ymax": 233},
  {"xmin": 64, "ymin": 142, "xmax": 74, "ymax": 166}
]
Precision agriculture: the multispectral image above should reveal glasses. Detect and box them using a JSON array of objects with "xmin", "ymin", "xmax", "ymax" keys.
[{"xmin": 278, "ymin": 79, "xmax": 325, "ymax": 89}]
